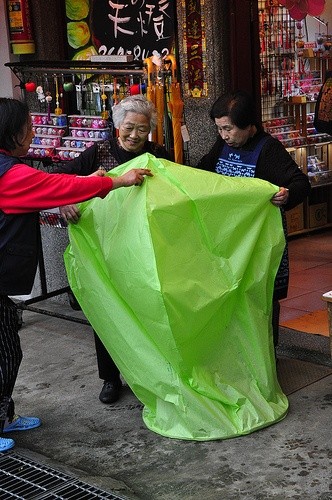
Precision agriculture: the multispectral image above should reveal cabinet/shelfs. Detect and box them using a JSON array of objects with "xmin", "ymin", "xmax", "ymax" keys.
[
  {"xmin": 296, "ymin": 71, "xmax": 323, "ymax": 103},
  {"xmin": 30, "ymin": 112, "xmax": 109, "ymax": 151},
  {"xmin": 268, "ymin": 113, "xmax": 332, "ymax": 236}
]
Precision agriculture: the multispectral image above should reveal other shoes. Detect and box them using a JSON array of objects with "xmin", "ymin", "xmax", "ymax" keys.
[
  {"xmin": 2, "ymin": 414, "xmax": 42, "ymax": 434},
  {"xmin": 99, "ymin": 378, "xmax": 122, "ymax": 404},
  {"xmin": 0, "ymin": 437, "xmax": 16, "ymax": 452}
]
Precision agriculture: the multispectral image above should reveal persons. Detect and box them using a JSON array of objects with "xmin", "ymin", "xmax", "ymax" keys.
[
  {"xmin": 314, "ymin": 75, "xmax": 332, "ymax": 136},
  {"xmin": 193, "ymin": 91, "xmax": 311, "ymax": 371},
  {"xmin": 59, "ymin": 94, "xmax": 170, "ymax": 404},
  {"xmin": 0, "ymin": 96, "xmax": 153, "ymax": 452}
]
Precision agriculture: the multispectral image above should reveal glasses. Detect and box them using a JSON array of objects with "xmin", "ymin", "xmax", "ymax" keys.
[{"xmin": 120, "ymin": 123, "xmax": 152, "ymax": 135}]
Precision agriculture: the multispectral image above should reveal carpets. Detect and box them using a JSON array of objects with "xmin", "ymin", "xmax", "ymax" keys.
[{"xmin": 276, "ymin": 357, "xmax": 332, "ymax": 396}]
